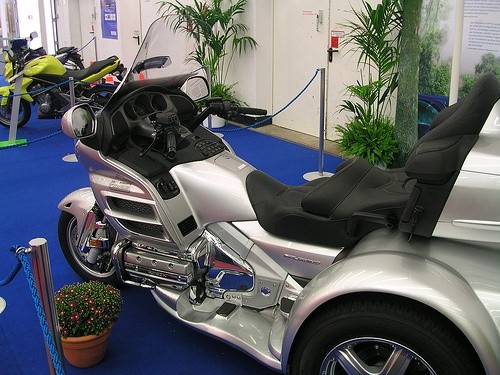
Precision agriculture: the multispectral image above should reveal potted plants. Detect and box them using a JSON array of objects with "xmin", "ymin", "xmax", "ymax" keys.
[
  {"xmin": 155, "ymin": 0, "xmax": 259, "ymax": 129},
  {"xmin": 333, "ymin": 0, "xmax": 405, "ymax": 173}
]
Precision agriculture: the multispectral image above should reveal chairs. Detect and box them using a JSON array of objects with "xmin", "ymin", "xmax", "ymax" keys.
[{"xmin": 300, "ymin": 71, "xmax": 500, "ymax": 241}]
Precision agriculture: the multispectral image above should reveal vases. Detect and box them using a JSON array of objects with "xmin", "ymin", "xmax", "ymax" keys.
[{"xmin": 60, "ymin": 322, "xmax": 114, "ymax": 368}]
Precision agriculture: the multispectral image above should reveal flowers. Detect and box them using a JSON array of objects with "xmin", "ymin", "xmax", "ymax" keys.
[{"xmin": 52, "ymin": 281, "xmax": 125, "ymax": 338}]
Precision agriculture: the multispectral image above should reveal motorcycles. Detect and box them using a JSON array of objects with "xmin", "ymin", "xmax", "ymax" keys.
[
  {"xmin": 0, "ymin": 31, "xmax": 135, "ymax": 128},
  {"xmin": 59, "ymin": 14, "xmax": 500, "ymax": 375}
]
